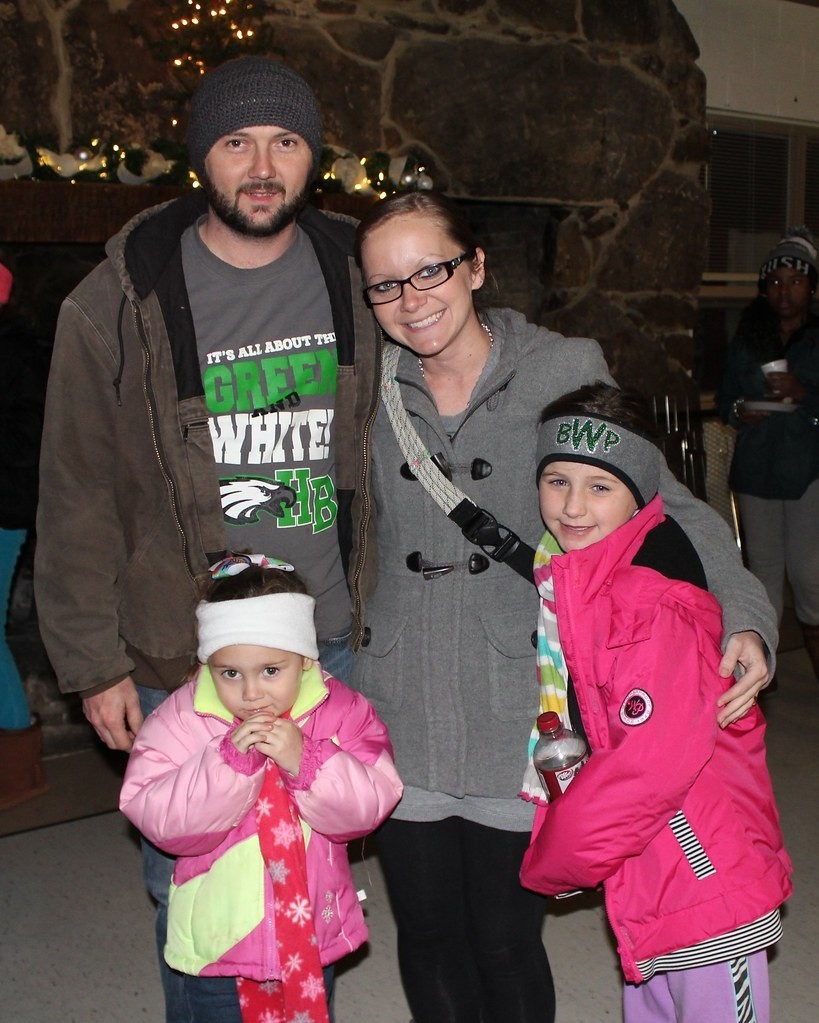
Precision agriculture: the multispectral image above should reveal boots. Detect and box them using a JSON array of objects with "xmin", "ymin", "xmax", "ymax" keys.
[{"xmin": 0, "ymin": 711, "xmax": 50, "ymax": 812}]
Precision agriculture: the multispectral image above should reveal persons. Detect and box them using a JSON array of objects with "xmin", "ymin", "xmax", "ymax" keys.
[
  {"xmin": 358, "ymin": 186, "xmax": 780, "ymax": 1023},
  {"xmin": 117, "ymin": 553, "xmax": 402, "ymax": 1023},
  {"xmin": 520, "ymin": 378, "xmax": 797, "ymax": 1023},
  {"xmin": 0, "ymin": 262, "xmax": 51, "ymax": 815},
  {"xmin": 34, "ymin": 55, "xmax": 388, "ymax": 1023},
  {"xmin": 721, "ymin": 221, "xmax": 818, "ymax": 697}
]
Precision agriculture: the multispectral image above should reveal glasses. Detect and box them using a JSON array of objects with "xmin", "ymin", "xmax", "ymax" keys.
[{"xmin": 362, "ymin": 251, "xmax": 476, "ymax": 309}]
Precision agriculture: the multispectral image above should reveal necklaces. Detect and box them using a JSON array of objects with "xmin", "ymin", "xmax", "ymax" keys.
[{"xmin": 418, "ymin": 322, "xmax": 494, "ymax": 375}]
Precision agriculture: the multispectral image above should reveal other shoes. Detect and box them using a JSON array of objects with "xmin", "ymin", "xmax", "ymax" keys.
[{"xmin": 758, "ymin": 673, "xmax": 780, "ymax": 707}]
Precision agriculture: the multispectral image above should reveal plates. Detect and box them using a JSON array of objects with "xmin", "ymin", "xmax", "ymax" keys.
[{"xmin": 742, "ymin": 402, "xmax": 798, "ymax": 415}]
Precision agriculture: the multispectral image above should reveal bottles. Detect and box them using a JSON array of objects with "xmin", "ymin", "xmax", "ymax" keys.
[{"xmin": 532, "ymin": 712, "xmax": 590, "ymax": 804}]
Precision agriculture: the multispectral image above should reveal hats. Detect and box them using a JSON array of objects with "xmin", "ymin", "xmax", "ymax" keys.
[
  {"xmin": 186, "ymin": 55, "xmax": 322, "ymax": 186},
  {"xmin": 758, "ymin": 224, "xmax": 819, "ymax": 285}
]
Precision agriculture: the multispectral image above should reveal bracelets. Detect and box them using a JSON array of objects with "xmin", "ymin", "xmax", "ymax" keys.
[{"xmin": 733, "ymin": 397, "xmax": 743, "ymax": 420}]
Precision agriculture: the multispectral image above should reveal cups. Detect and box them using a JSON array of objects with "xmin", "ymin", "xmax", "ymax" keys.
[{"xmin": 761, "ymin": 359, "xmax": 788, "ymax": 394}]
